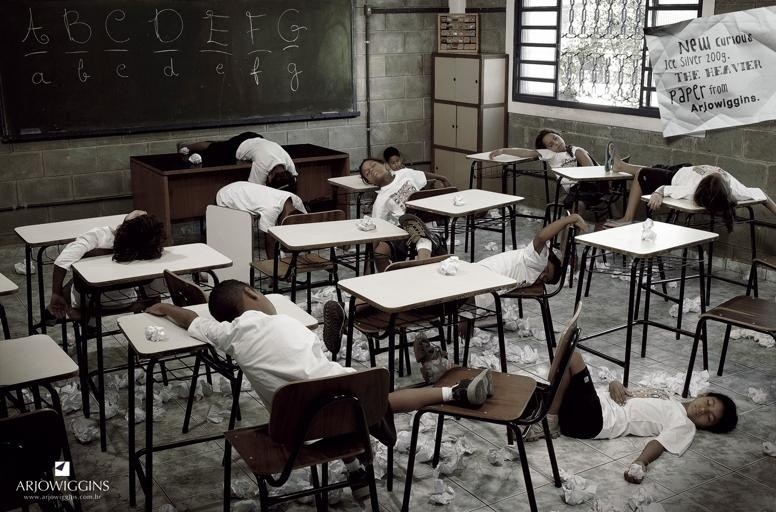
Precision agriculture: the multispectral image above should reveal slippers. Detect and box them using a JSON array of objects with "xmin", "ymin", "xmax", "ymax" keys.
[
  {"xmin": 605, "ymin": 142, "xmax": 616, "ymax": 172},
  {"xmin": 602, "ymin": 220, "xmax": 623, "ymax": 229}
]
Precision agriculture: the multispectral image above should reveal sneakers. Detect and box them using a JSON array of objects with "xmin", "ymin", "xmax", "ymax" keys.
[
  {"xmin": 512, "ymin": 421, "xmax": 560, "ymax": 442},
  {"xmin": 323, "ymin": 300, "xmax": 345, "ymax": 353},
  {"xmin": 45, "ymin": 311, "xmax": 57, "ymax": 327},
  {"xmin": 452, "ymin": 369, "xmax": 495, "ymax": 408},
  {"xmin": 399, "ymin": 214, "xmax": 439, "ymax": 249},
  {"xmin": 348, "ymin": 471, "xmax": 370, "ymax": 500},
  {"xmin": 415, "ymin": 333, "xmax": 448, "ymax": 384}
]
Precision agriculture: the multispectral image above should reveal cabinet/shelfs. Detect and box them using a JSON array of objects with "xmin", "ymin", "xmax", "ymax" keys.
[{"xmin": 431, "ymin": 54, "xmax": 507, "ymax": 194}]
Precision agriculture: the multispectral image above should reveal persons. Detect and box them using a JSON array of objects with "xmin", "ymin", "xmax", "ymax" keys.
[
  {"xmin": 603, "ymin": 140, "xmax": 776, "ymax": 228},
  {"xmin": 48, "ymin": 209, "xmax": 164, "ymax": 337},
  {"xmin": 357, "ymin": 157, "xmax": 451, "ymax": 273},
  {"xmin": 488, "ymin": 128, "xmax": 620, "ymax": 236},
  {"xmin": 412, "ymin": 331, "xmax": 738, "ymax": 485},
  {"xmin": 175, "ymin": 130, "xmax": 299, "ymax": 194},
  {"xmin": 383, "ymin": 146, "xmax": 406, "ymax": 172},
  {"xmin": 139, "ymin": 277, "xmax": 495, "ymax": 502},
  {"xmin": 214, "ymin": 180, "xmax": 309, "ymax": 289},
  {"xmin": 322, "ymin": 213, "xmax": 591, "ymax": 354}
]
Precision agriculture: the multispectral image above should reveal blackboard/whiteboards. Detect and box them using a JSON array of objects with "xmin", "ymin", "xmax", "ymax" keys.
[{"xmin": 0, "ymin": 0, "xmax": 360, "ymax": 144}]
